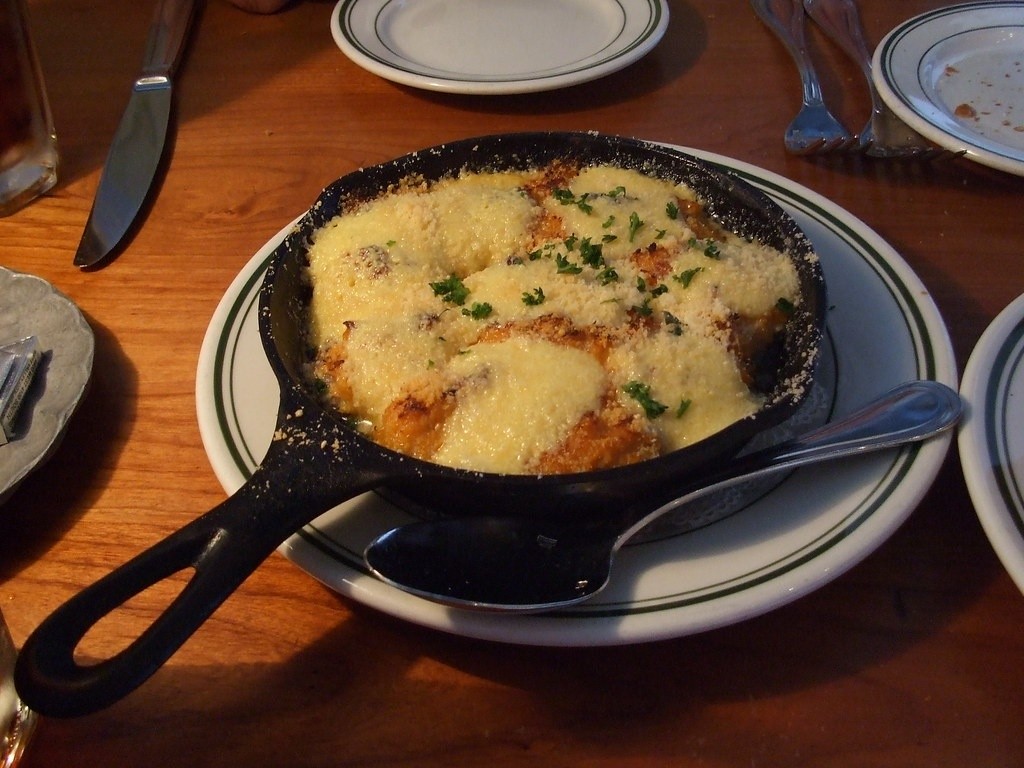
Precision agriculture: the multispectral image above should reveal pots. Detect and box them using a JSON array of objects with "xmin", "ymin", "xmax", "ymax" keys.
[{"xmin": 10, "ymin": 128, "xmax": 833, "ymax": 723}]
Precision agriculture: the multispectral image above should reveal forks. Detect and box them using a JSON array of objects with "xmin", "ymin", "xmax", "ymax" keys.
[{"xmin": 746, "ymin": 1, "xmax": 969, "ymax": 176}]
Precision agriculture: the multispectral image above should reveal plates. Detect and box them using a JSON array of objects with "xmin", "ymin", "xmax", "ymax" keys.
[
  {"xmin": 871, "ymin": 2, "xmax": 1024, "ymax": 180},
  {"xmin": 958, "ymin": 286, "xmax": 1024, "ymax": 593},
  {"xmin": 329, "ymin": 0, "xmax": 672, "ymax": 99},
  {"xmin": 0, "ymin": 264, "xmax": 96, "ymax": 499},
  {"xmin": 193, "ymin": 138, "xmax": 961, "ymax": 650}
]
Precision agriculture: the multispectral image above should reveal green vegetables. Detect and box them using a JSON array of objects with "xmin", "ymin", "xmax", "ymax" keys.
[{"xmin": 426, "ymin": 187, "xmax": 722, "ymax": 419}]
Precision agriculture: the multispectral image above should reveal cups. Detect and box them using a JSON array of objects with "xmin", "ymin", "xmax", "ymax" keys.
[
  {"xmin": 0, "ymin": 606, "xmax": 39, "ymax": 767},
  {"xmin": 0, "ymin": 0, "xmax": 61, "ymax": 220}
]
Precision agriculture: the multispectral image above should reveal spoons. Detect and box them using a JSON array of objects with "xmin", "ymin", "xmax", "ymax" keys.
[{"xmin": 359, "ymin": 380, "xmax": 973, "ymax": 613}]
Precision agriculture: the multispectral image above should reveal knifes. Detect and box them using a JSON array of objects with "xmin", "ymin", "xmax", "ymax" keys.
[{"xmin": 73, "ymin": 0, "xmax": 199, "ymax": 268}]
